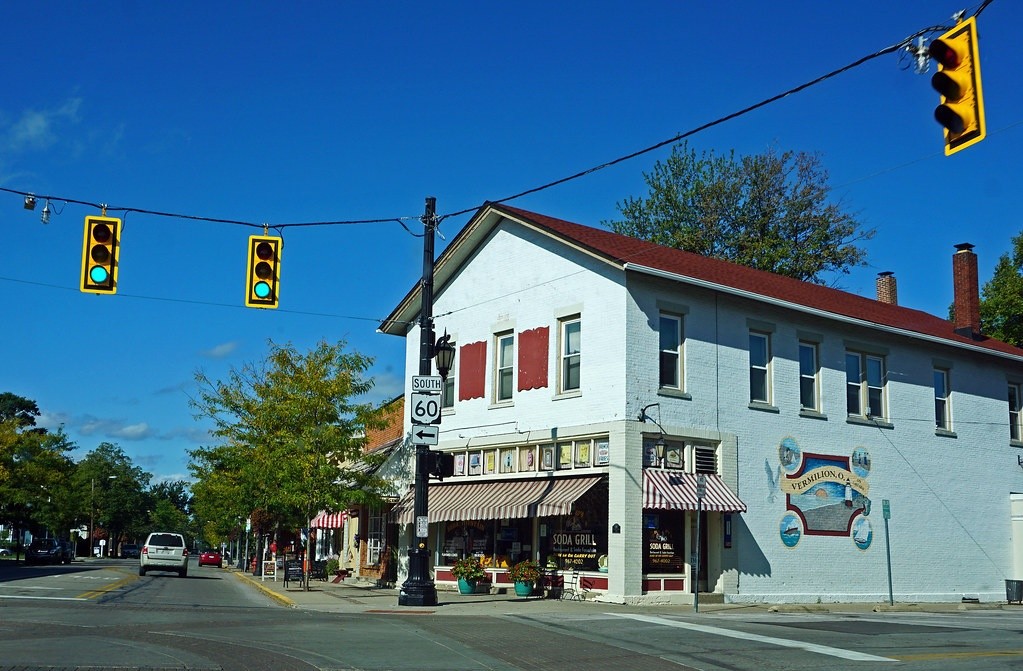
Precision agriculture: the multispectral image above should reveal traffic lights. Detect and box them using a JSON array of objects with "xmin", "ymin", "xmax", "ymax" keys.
[
  {"xmin": 80, "ymin": 216, "xmax": 121, "ymax": 294},
  {"xmin": 928, "ymin": 14, "xmax": 987, "ymax": 157},
  {"xmin": 245, "ymin": 235, "xmax": 282, "ymax": 309}
]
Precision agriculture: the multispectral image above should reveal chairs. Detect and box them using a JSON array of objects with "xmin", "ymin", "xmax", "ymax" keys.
[{"xmin": 550, "ymin": 565, "xmax": 581, "ymax": 602}]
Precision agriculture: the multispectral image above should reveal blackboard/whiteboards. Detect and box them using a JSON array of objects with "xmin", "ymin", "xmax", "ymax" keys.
[{"xmin": 284, "ymin": 559, "xmax": 304, "ymax": 582}]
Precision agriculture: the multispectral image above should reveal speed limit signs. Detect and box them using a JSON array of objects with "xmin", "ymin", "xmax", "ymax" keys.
[{"xmin": 411, "ymin": 392, "xmax": 442, "ymax": 424}]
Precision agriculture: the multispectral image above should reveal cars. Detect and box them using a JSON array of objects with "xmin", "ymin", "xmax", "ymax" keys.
[
  {"xmin": 198, "ymin": 549, "xmax": 224, "ymax": 568},
  {"xmin": 24, "ymin": 539, "xmax": 72, "ymax": 566},
  {"xmin": 0, "ymin": 549, "xmax": 13, "ymax": 557},
  {"xmin": 139, "ymin": 533, "xmax": 189, "ymax": 577},
  {"xmin": 120, "ymin": 544, "xmax": 140, "ymax": 559}
]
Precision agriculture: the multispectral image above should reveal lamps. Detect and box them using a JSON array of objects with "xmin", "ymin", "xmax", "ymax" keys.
[
  {"xmin": 638, "ymin": 403, "xmax": 669, "ymax": 460},
  {"xmin": 41, "ymin": 198, "xmax": 68, "ymax": 224}
]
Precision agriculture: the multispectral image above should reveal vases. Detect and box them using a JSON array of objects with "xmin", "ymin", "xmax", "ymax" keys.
[
  {"xmin": 514, "ymin": 579, "xmax": 534, "ymax": 596},
  {"xmin": 457, "ymin": 578, "xmax": 476, "ymax": 594}
]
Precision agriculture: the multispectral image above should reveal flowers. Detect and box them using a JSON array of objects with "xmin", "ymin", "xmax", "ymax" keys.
[
  {"xmin": 507, "ymin": 559, "xmax": 544, "ymax": 583},
  {"xmin": 449, "ymin": 556, "xmax": 487, "ymax": 581}
]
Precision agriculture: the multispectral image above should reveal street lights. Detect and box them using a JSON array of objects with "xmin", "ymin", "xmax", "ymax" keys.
[
  {"xmin": 398, "ymin": 325, "xmax": 456, "ymax": 602},
  {"xmin": 90, "ymin": 475, "xmax": 117, "ymax": 557}
]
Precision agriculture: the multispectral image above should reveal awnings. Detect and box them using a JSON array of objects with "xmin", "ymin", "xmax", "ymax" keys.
[
  {"xmin": 310, "ymin": 510, "xmax": 348, "ymax": 529},
  {"xmin": 388, "ymin": 476, "xmax": 601, "ymax": 524},
  {"xmin": 641, "ymin": 467, "xmax": 747, "ymax": 514}
]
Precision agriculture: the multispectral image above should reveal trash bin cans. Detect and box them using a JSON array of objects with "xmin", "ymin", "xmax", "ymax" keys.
[{"xmin": 1005, "ymin": 580, "xmax": 1023, "ymax": 604}]
